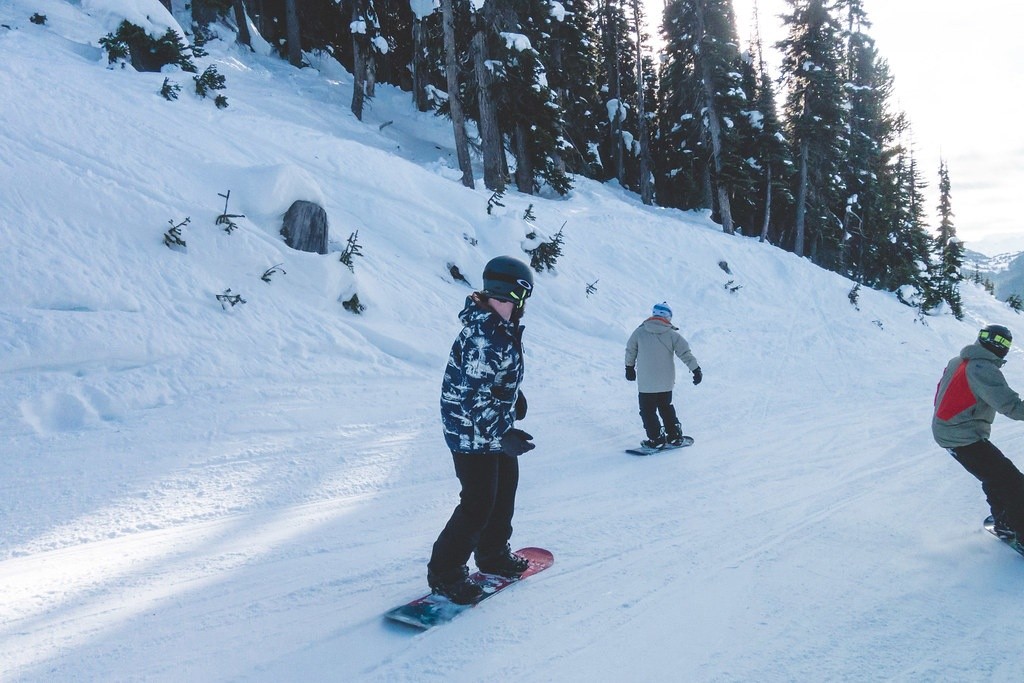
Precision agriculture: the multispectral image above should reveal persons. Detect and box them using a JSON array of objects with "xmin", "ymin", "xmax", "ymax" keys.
[
  {"xmin": 931, "ymin": 324, "xmax": 1024, "ymax": 549},
  {"xmin": 426, "ymin": 255, "xmax": 535, "ymax": 604},
  {"xmin": 624, "ymin": 302, "xmax": 702, "ymax": 450}
]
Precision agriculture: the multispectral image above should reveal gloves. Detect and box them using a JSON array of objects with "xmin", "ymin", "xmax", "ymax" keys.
[
  {"xmin": 692, "ymin": 366, "xmax": 703, "ymax": 385},
  {"xmin": 515, "ymin": 390, "xmax": 528, "ymax": 420},
  {"xmin": 625, "ymin": 365, "xmax": 635, "ymax": 381},
  {"xmin": 498, "ymin": 429, "xmax": 536, "ymax": 456}
]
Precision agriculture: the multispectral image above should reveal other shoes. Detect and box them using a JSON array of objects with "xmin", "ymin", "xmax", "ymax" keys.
[
  {"xmin": 994, "ymin": 522, "xmax": 1016, "ymax": 536},
  {"xmin": 1016, "ymin": 539, "xmax": 1024, "ymax": 550},
  {"xmin": 640, "ymin": 438, "xmax": 665, "ymax": 450},
  {"xmin": 665, "ymin": 432, "xmax": 683, "ymax": 445}
]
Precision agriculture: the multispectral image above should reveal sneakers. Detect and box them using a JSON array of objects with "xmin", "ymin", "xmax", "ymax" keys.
[
  {"xmin": 475, "ymin": 548, "xmax": 531, "ymax": 577},
  {"xmin": 427, "ymin": 566, "xmax": 483, "ymax": 603}
]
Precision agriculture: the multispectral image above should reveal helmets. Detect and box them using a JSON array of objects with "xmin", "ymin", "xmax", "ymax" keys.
[
  {"xmin": 653, "ymin": 303, "xmax": 673, "ymax": 322},
  {"xmin": 482, "ymin": 256, "xmax": 534, "ymax": 299},
  {"xmin": 978, "ymin": 324, "xmax": 1012, "ymax": 357}
]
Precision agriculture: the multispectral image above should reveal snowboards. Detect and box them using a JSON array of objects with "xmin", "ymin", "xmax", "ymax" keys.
[
  {"xmin": 381, "ymin": 544, "xmax": 555, "ymax": 633},
  {"xmin": 983, "ymin": 514, "xmax": 1024, "ymax": 557},
  {"xmin": 625, "ymin": 435, "xmax": 695, "ymax": 457}
]
